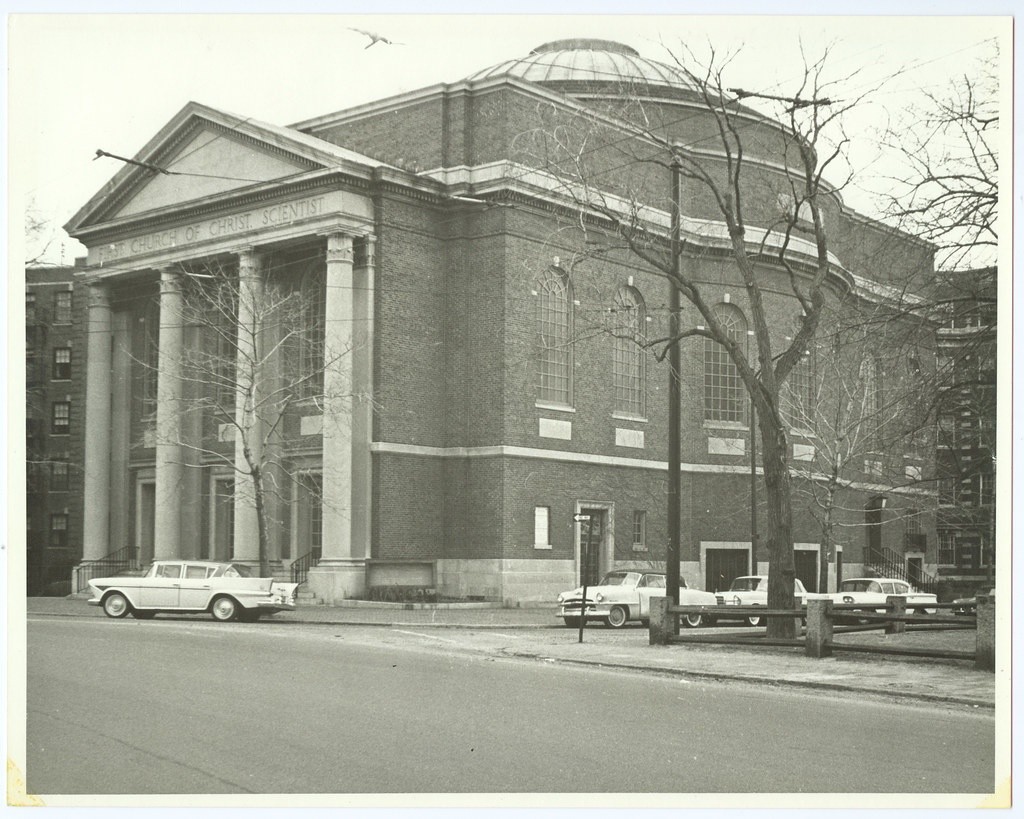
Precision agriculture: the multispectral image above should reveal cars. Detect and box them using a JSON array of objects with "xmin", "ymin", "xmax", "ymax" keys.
[
  {"xmin": 701, "ymin": 575, "xmax": 833, "ymax": 629},
  {"xmin": 556, "ymin": 569, "xmax": 722, "ymax": 629},
  {"xmin": 86, "ymin": 561, "xmax": 299, "ymax": 622}
]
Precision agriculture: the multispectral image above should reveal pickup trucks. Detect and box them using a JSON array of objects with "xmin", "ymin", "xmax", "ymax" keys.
[{"xmin": 829, "ymin": 578, "xmax": 938, "ymax": 624}]
